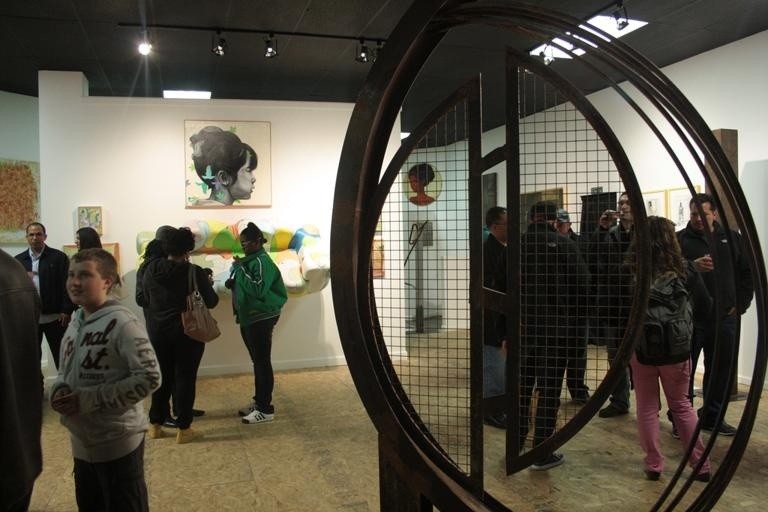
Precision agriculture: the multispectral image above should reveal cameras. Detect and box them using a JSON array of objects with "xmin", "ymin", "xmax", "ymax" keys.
[{"xmin": 607, "ymin": 211, "xmax": 621, "ymax": 220}]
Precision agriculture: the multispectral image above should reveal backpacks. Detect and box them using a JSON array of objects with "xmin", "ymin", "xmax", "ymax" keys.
[
  {"xmin": 626, "ymin": 261, "xmax": 695, "ymax": 367},
  {"xmin": 180, "ymin": 290, "xmax": 221, "ymax": 343}
]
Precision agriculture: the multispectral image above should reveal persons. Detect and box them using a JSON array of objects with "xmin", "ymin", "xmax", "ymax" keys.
[
  {"xmin": 0, "ymin": 244, "xmax": 51, "ymax": 512},
  {"xmin": 407, "ymin": 164, "xmax": 438, "ymax": 205},
  {"xmin": 189, "ymin": 126, "xmax": 258, "ymax": 206},
  {"xmin": 225, "ymin": 223, "xmax": 287, "ymax": 425},
  {"xmin": 46, "ymin": 251, "xmax": 163, "ymax": 511},
  {"xmin": 147, "ymin": 229, "xmax": 218, "ymax": 444},
  {"xmin": 12, "ymin": 223, "xmax": 72, "ymax": 375},
  {"xmin": 136, "ymin": 227, "xmax": 213, "ymax": 427},
  {"xmin": 74, "ymin": 227, "xmax": 103, "ymax": 256}
]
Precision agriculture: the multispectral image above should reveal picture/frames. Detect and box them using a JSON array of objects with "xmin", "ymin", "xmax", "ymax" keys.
[
  {"xmin": 62, "ymin": 240, "xmax": 122, "ymax": 281},
  {"xmin": 668, "ymin": 184, "xmax": 701, "ymax": 231},
  {"xmin": 638, "ymin": 189, "xmax": 667, "ymax": 222},
  {"xmin": 182, "ymin": 119, "xmax": 274, "ymax": 210}
]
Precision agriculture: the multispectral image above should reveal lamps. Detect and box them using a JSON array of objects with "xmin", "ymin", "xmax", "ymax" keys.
[
  {"xmin": 537, "ymin": 2, "xmax": 634, "ymax": 68},
  {"xmin": 133, "ymin": 31, "xmax": 386, "ymax": 66}
]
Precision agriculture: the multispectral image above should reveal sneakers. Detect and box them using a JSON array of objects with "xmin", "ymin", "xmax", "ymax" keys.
[
  {"xmin": 238, "ymin": 402, "xmax": 264, "ymax": 415},
  {"xmin": 713, "ymin": 418, "xmax": 738, "ymax": 437},
  {"xmin": 150, "ymin": 423, "xmax": 173, "ymax": 439},
  {"xmin": 241, "ymin": 410, "xmax": 275, "ymax": 424},
  {"xmin": 530, "ymin": 452, "xmax": 567, "ymax": 471},
  {"xmin": 597, "ymin": 405, "xmax": 629, "ymax": 419},
  {"xmin": 176, "ymin": 427, "xmax": 200, "ymax": 444}
]
